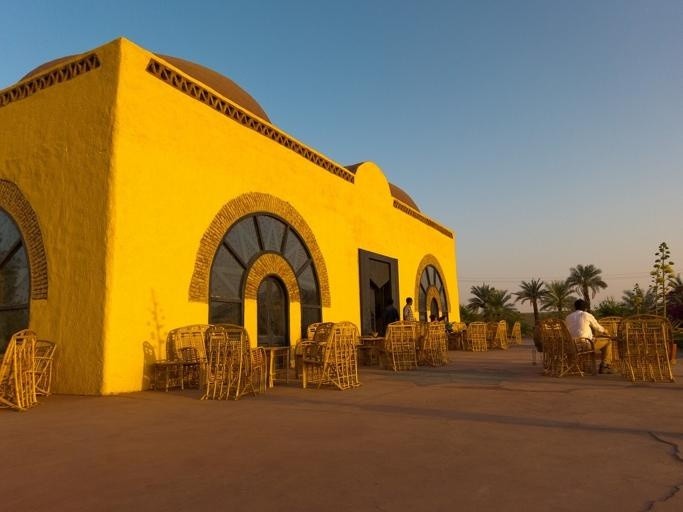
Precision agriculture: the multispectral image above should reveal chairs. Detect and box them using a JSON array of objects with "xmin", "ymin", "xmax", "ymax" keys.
[
  {"xmin": 532, "ymin": 313, "xmax": 676, "ymax": 384},
  {"xmin": 0, "ymin": 328, "xmax": 57, "ymax": 413},
  {"xmin": 152, "ymin": 322, "xmax": 268, "ymax": 401},
  {"xmin": 294, "ymin": 320, "xmax": 362, "ymax": 392},
  {"xmin": 376, "ymin": 319, "xmax": 521, "ymax": 373}
]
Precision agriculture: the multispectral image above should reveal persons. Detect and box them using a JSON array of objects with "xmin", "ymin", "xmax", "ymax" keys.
[
  {"xmin": 564, "ymin": 298, "xmax": 618, "ymax": 374},
  {"xmin": 403, "ymin": 297, "xmax": 414, "ymax": 322},
  {"xmin": 380, "ymin": 298, "xmax": 399, "ymax": 332}
]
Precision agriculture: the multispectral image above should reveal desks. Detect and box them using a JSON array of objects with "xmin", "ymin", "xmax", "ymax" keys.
[
  {"xmin": 359, "ymin": 335, "xmax": 386, "ymax": 370},
  {"xmin": 238, "ymin": 345, "xmax": 292, "ymax": 389}
]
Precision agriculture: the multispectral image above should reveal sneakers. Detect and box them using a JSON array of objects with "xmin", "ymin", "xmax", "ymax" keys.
[{"xmin": 599, "ymin": 363, "xmax": 618, "ymax": 374}]
